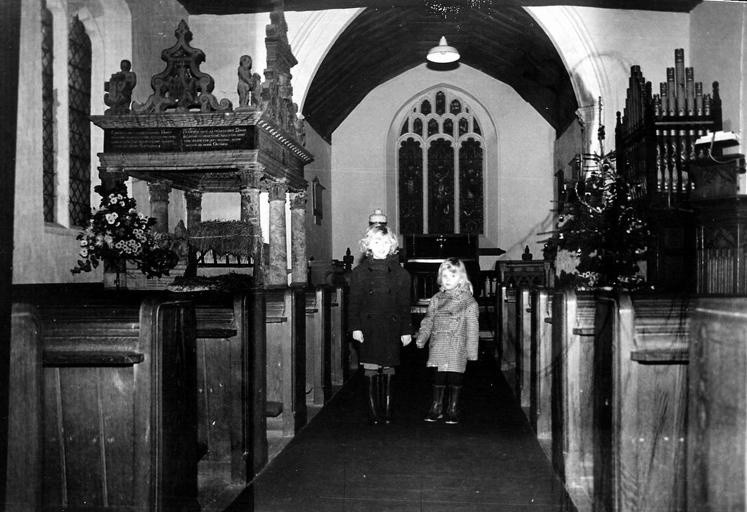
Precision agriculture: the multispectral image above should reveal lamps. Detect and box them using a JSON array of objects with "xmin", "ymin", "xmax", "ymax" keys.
[
  {"xmin": 692, "ymin": 129, "xmax": 746, "ymax": 174},
  {"xmin": 426, "ymin": 35, "xmax": 460, "ymax": 64}
]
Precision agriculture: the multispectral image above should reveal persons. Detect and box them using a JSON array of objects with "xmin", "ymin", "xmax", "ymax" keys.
[
  {"xmin": 103, "ymin": 60, "xmax": 136, "ymax": 107},
  {"xmin": 415, "ymin": 256, "xmax": 481, "ymax": 425},
  {"xmin": 346, "ymin": 221, "xmax": 416, "ymax": 423},
  {"xmin": 237, "ymin": 55, "xmax": 256, "ymax": 107}
]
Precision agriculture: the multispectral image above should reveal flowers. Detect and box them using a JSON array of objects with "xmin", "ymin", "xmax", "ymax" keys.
[
  {"xmin": 69, "ymin": 179, "xmax": 177, "ymax": 286},
  {"xmin": 543, "ymin": 175, "xmax": 655, "ymax": 295}
]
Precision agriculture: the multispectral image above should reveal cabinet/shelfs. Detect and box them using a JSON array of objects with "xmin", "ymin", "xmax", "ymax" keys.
[{"xmin": 693, "ymin": 194, "xmax": 747, "ymax": 295}]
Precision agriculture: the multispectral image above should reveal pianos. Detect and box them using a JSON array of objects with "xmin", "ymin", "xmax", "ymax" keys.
[{"xmin": 399, "ymin": 233, "xmax": 483, "ymax": 303}]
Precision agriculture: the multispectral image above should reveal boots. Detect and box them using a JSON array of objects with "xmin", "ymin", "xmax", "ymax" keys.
[
  {"xmin": 380, "ymin": 373, "xmax": 394, "ymax": 426},
  {"xmin": 365, "ymin": 374, "xmax": 381, "ymax": 426},
  {"xmin": 424, "ymin": 383, "xmax": 447, "ymax": 423},
  {"xmin": 445, "ymin": 385, "xmax": 464, "ymax": 425}
]
